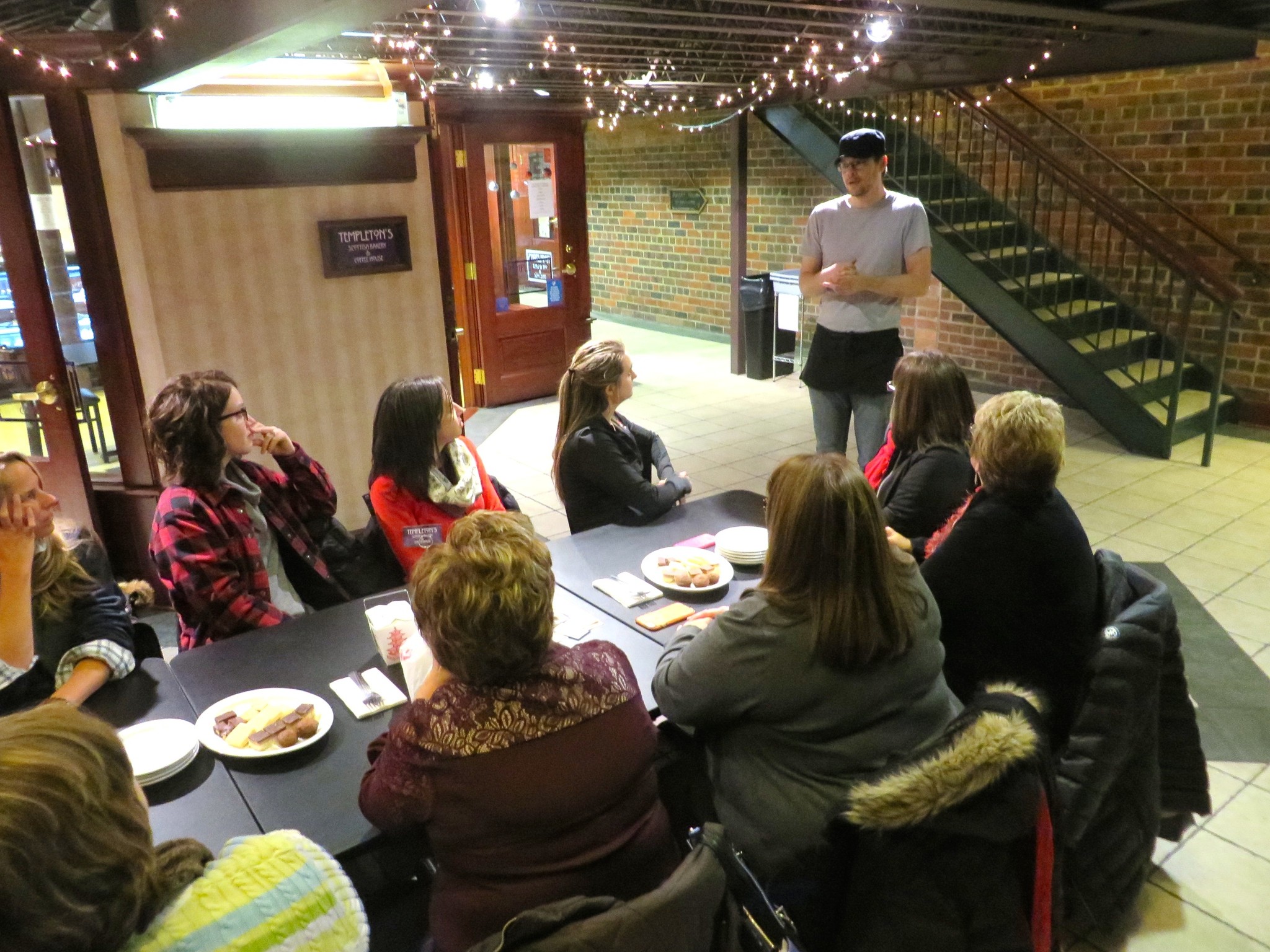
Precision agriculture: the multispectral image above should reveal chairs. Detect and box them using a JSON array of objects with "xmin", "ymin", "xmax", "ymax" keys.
[
  {"xmin": 461, "ymin": 552, "xmax": 1213, "ymax": 952},
  {"xmin": 10, "ymin": 353, "xmax": 109, "ymax": 464}
]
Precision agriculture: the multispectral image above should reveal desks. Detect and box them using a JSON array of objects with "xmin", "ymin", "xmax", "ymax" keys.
[
  {"xmin": 547, "ymin": 488, "xmax": 766, "ymax": 649},
  {"xmin": 767, "ymin": 270, "xmax": 804, "ymax": 390},
  {"xmin": 168, "ymin": 584, "xmax": 668, "ymax": 866},
  {"xmin": 77, "ymin": 658, "xmax": 264, "ymax": 860}
]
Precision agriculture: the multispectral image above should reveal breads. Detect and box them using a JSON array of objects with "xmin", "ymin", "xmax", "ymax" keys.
[
  {"xmin": 675, "ymin": 571, "xmax": 719, "ymax": 588},
  {"xmin": 277, "ymin": 716, "xmax": 319, "ymax": 746}
]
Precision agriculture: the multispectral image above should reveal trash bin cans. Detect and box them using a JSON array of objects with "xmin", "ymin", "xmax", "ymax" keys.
[{"xmin": 739, "ymin": 272, "xmax": 796, "ymax": 380}]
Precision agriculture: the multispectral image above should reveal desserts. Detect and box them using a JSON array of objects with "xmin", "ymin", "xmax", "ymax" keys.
[
  {"xmin": 658, "ymin": 555, "xmax": 720, "ymax": 582},
  {"xmin": 212, "ymin": 700, "xmax": 315, "ymax": 752}
]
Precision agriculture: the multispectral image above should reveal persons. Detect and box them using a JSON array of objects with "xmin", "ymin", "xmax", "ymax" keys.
[
  {"xmin": 799, "ymin": 127, "xmax": 932, "ymax": 472},
  {"xmin": 148, "ymin": 369, "xmax": 369, "ymax": 655},
  {"xmin": 863, "ymin": 353, "xmax": 976, "ymax": 566},
  {"xmin": 359, "ymin": 510, "xmax": 680, "ymax": 952},
  {"xmin": 0, "ymin": 682, "xmax": 374, "ymax": 952},
  {"xmin": 652, "ymin": 454, "xmax": 967, "ymax": 952},
  {"xmin": 368, "ymin": 374, "xmax": 507, "ymax": 584},
  {"xmin": 550, "ymin": 338, "xmax": 691, "ymax": 537},
  {"xmin": 884, "ymin": 391, "xmax": 1103, "ymax": 768},
  {"xmin": 0, "ymin": 450, "xmax": 137, "ymax": 718}
]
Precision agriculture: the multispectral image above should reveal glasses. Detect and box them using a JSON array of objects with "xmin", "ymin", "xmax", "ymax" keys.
[
  {"xmin": 837, "ymin": 157, "xmax": 875, "ymax": 174},
  {"xmin": 887, "ymin": 381, "xmax": 896, "ymax": 393},
  {"xmin": 219, "ymin": 408, "xmax": 250, "ymax": 422}
]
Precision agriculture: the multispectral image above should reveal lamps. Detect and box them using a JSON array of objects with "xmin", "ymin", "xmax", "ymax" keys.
[{"xmin": 487, "ymin": 145, "xmax": 521, "ymax": 200}]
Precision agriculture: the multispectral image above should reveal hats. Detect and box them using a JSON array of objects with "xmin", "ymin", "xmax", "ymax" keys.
[{"xmin": 834, "ymin": 128, "xmax": 887, "ymax": 166}]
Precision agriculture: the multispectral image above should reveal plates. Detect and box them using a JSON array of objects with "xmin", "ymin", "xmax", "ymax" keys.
[
  {"xmin": 117, "ymin": 717, "xmax": 200, "ymax": 788},
  {"xmin": 715, "ymin": 526, "xmax": 770, "ymax": 565},
  {"xmin": 641, "ymin": 546, "xmax": 735, "ymax": 594},
  {"xmin": 195, "ymin": 687, "xmax": 334, "ymax": 759}
]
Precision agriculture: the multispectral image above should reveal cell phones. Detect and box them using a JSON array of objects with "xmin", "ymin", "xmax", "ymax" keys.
[
  {"xmin": 636, "ymin": 602, "xmax": 695, "ymax": 631},
  {"xmin": 674, "ymin": 533, "xmax": 715, "ymax": 548}
]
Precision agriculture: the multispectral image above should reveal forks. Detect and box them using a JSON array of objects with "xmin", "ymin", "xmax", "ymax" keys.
[{"xmin": 348, "ymin": 670, "xmax": 385, "ymax": 710}]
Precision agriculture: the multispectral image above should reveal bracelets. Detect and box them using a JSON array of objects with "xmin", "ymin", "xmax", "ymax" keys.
[{"xmin": 38, "ymin": 697, "xmax": 77, "ymax": 711}]
